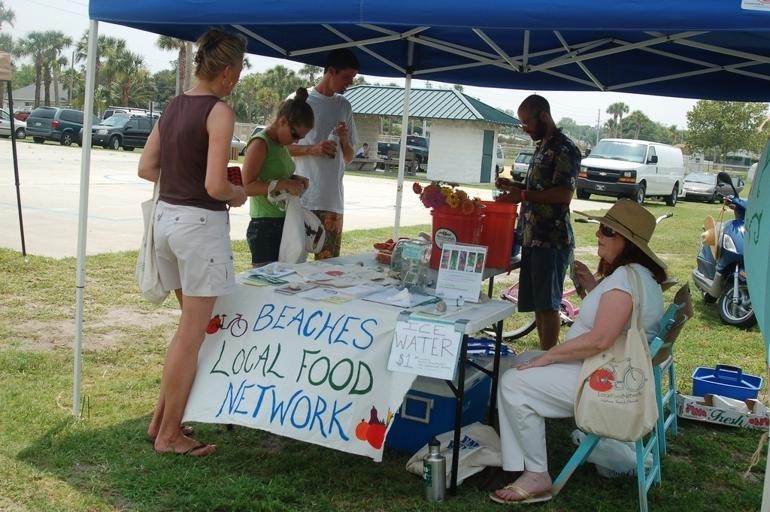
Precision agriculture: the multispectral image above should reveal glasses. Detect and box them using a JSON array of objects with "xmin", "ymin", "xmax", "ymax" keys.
[
  {"xmin": 290, "ymin": 128, "xmax": 305, "ymax": 142},
  {"xmin": 519, "ymin": 114, "xmax": 537, "ymax": 127},
  {"xmin": 596, "ymin": 222, "xmax": 619, "ymax": 237}
]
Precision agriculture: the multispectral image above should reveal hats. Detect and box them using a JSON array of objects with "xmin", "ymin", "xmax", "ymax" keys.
[
  {"xmin": 574, "ymin": 198, "xmax": 666, "ymax": 269},
  {"xmin": 701, "ymin": 214, "xmax": 718, "ymax": 260}
]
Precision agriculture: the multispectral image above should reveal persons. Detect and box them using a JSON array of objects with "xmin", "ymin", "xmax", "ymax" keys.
[
  {"xmin": 356, "ymin": 143, "xmax": 370, "ymax": 170},
  {"xmin": 493, "ymin": 94, "xmax": 581, "ymax": 349},
  {"xmin": 239, "ymin": 87, "xmax": 315, "ymax": 268},
  {"xmin": 282, "ymin": 47, "xmax": 359, "ymax": 262},
  {"xmin": 135, "ymin": 27, "xmax": 246, "ymax": 458},
  {"xmin": 487, "ymin": 199, "xmax": 668, "ymax": 505}
]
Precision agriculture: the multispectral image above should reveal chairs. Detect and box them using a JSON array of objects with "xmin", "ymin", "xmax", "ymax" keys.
[{"xmin": 549, "ymin": 301, "xmax": 681, "ymax": 511}]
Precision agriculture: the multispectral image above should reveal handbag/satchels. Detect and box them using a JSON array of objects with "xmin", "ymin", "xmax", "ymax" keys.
[
  {"xmin": 136, "ymin": 168, "xmax": 172, "ymax": 304},
  {"xmin": 267, "ymin": 179, "xmax": 326, "ymax": 264},
  {"xmin": 408, "ymin": 418, "xmax": 507, "ymax": 487},
  {"xmin": 572, "ymin": 264, "xmax": 659, "ymax": 439}
]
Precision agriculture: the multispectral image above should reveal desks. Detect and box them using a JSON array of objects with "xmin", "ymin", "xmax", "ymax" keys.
[
  {"xmin": 320, "ymin": 252, "xmax": 523, "ymax": 300},
  {"xmin": 222, "ymin": 260, "xmax": 517, "ymax": 499}
]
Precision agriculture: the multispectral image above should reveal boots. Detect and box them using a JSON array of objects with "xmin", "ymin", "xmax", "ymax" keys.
[{"xmin": 155, "ymin": 439, "xmax": 218, "ymax": 458}]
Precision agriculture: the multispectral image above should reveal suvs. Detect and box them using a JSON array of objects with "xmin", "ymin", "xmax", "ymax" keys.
[
  {"xmin": 26, "ymin": 105, "xmax": 102, "ymax": 146},
  {"xmin": 509, "ymin": 151, "xmax": 535, "ymax": 181},
  {"xmin": 102, "ymin": 106, "xmax": 148, "ymax": 121},
  {"xmin": 400, "ymin": 135, "xmax": 429, "ymax": 163},
  {"xmin": 78, "ymin": 112, "xmax": 161, "ymax": 150}
]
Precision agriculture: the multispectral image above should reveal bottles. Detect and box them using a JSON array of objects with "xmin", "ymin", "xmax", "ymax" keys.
[
  {"xmin": 423, "ymin": 435, "xmax": 446, "ymax": 504},
  {"xmin": 325, "ymin": 126, "xmax": 341, "ymax": 159}
]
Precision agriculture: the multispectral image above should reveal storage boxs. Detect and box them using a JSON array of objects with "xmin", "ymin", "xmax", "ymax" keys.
[
  {"xmin": 693, "ymin": 366, "xmax": 762, "ymax": 406},
  {"xmin": 385, "ymin": 357, "xmax": 492, "ymax": 461}
]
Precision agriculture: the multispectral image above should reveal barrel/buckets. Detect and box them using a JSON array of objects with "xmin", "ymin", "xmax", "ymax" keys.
[
  {"xmin": 430, "ymin": 202, "xmax": 488, "ymax": 271},
  {"xmin": 478, "ymin": 200, "xmax": 521, "ymax": 267}
]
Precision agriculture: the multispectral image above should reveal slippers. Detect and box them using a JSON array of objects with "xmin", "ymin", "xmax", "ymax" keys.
[
  {"xmin": 490, "ymin": 483, "xmax": 552, "ymax": 504},
  {"xmin": 148, "ymin": 424, "xmax": 195, "ymax": 441}
]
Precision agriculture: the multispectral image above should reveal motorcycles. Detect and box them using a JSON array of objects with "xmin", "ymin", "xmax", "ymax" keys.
[{"xmin": 692, "ymin": 191, "xmax": 758, "ymax": 331}]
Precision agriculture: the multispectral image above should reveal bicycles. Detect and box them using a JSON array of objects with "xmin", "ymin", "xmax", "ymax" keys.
[{"xmin": 478, "ymin": 210, "xmax": 674, "ymax": 341}]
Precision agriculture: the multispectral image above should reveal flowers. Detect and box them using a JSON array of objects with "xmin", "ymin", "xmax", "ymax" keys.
[{"xmin": 411, "ymin": 179, "xmax": 482, "ymax": 213}]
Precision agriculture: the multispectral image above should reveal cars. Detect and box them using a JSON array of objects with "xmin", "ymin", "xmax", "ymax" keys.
[
  {"xmin": 378, "ymin": 142, "xmax": 400, "ymax": 171},
  {"xmin": 0, "ymin": 108, "xmax": 28, "ymax": 139},
  {"xmin": 12, "ymin": 110, "xmax": 31, "ymax": 122},
  {"xmin": 683, "ymin": 172, "xmax": 744, "ymax": 204},
  {"xmin": 231, "ymin": 136, "xmax": 247, "ymax": 156}
]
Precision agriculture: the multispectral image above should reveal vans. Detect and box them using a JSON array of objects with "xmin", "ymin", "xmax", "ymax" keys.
[
  {"xmin": 495, "ymin": 145, "xmax": 504, "ymax": 178},
  {"xmin": 576, "ymin": 138, "xmax": 686, "ymax": 206}
]
